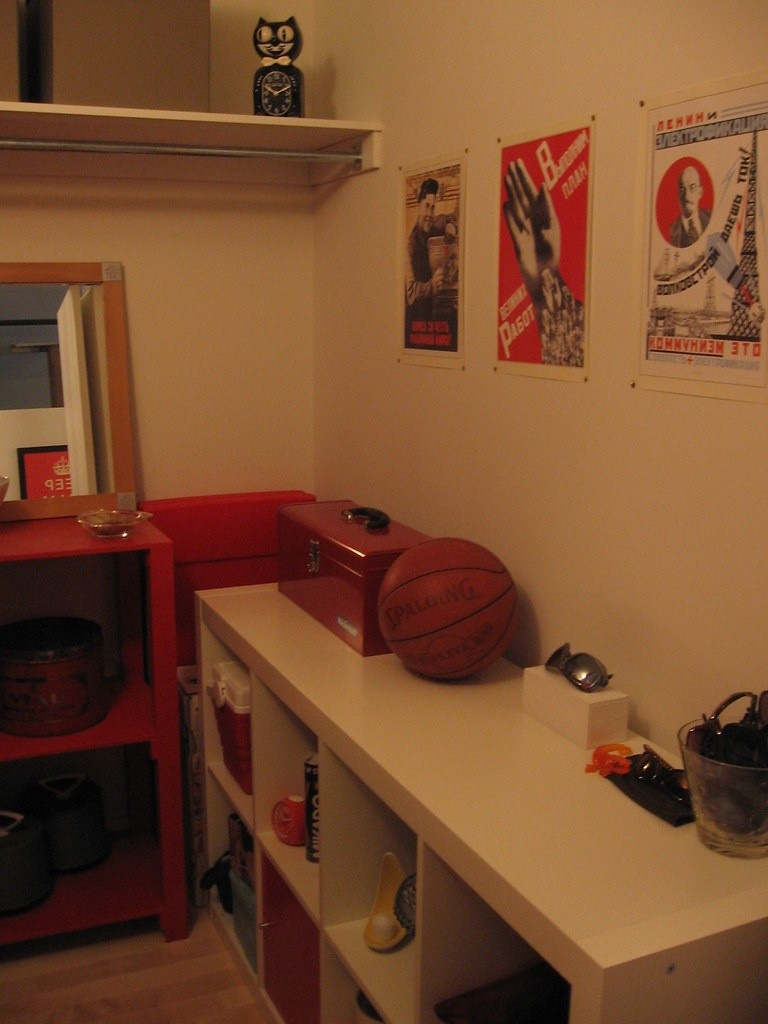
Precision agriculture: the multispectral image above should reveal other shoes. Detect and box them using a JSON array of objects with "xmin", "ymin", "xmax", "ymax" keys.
[{"xmin": 364, "ymin": 848, "xmax": 413, "ymax": 953}]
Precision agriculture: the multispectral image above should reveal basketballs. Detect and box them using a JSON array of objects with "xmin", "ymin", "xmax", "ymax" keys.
[{"xmin": 376, "ymin": 538, "xmax": 518, "ymax": 682}]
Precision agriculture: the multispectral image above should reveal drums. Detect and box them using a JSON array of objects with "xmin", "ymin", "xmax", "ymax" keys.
[{"xmin": 1, "ymin": 615, "xmax": 111, "ymax": 739}]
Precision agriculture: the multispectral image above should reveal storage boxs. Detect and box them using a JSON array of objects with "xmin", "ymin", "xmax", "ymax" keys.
[
  {"xmin": 208, "ymin": 659, "xmax": 252, "ymax": 797},
  {"xmin": 0, "ymin": 0, "xmax": 212, "ymax": 112}
]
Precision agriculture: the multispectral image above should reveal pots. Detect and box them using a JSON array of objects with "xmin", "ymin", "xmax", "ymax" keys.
[{"xmin": 0, "ymin": 616, "xmax": 111, "ymax": 737}]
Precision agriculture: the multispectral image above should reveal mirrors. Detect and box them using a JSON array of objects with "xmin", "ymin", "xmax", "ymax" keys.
[{"xmin": 0, "ymin": 261, "xmax": 138, "ymax": 522}]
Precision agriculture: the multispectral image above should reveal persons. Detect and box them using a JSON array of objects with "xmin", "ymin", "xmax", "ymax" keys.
[
  {"xmin": 503, "ymin": 158, "xmax": 581, "ymax": 332},
  {"xmin": 404, "ymin": 178, "xmax": 459, "ymax": 306},
  {"xmin": 669, "ymin": 166, "xmax": 712, "ymax": 248}
]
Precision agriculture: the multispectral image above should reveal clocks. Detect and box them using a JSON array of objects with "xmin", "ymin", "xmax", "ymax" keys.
[{"xmin": 253, "ymin": 15, "xmax": 304, "ymax": 117}]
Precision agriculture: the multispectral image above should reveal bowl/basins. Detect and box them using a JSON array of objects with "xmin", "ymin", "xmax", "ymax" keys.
[
  {"xmin": 75, "ymin": 509, "xmax": 153, "ymax": 538},
  {"xmin": 678, "ymin": 716, "xmax": 768, "ymax": 859}
]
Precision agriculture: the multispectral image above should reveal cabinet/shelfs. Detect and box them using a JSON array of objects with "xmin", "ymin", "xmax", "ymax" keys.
[
  {"xmin": 194, "ymin": 583, "xmax": 767, "ymax": 1024},
  {"xmin": 0, "ymin": 516, "xmax": 188, "ymax": 946}
]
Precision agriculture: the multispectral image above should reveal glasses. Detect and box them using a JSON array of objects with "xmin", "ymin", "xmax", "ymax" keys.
[
  {"xmin": 635, "ymin": 750, "xmax": 687, "ymax": 802},
  {"xmin": 687, "ymin": 690, "xmax": 768, "ymax": 835},
  {"xmin": 545, "ymin": 642, "xmax": 613, "ymax": 693}
]
